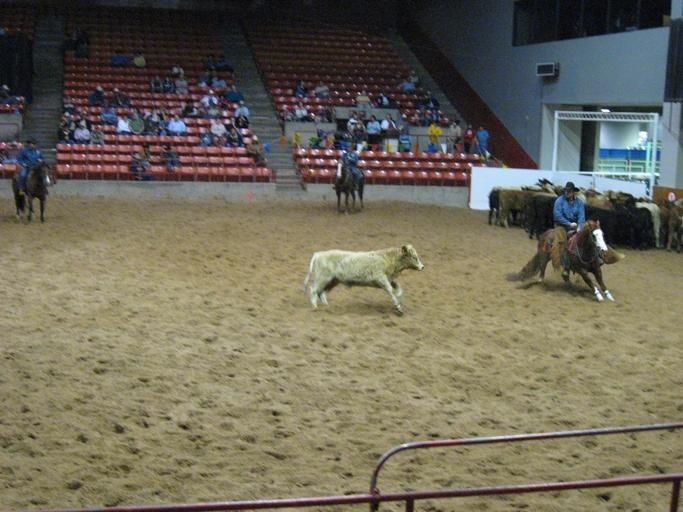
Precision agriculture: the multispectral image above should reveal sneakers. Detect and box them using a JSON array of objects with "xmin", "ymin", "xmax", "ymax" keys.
[{"xmin": 562, "ymin": 270, "xmax": 570, "ymax": 281}]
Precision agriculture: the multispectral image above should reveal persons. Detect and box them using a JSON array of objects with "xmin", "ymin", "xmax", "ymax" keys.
[
  {"xmin": 58, "ymin": 43, "xmax": 263, "ymax": 178},
  {"xmin": 291, "ymin": 72, "xmax": 495, "ymax": 182},
  {"xmin": 0, "ymin": 77, "xmax": 41, "ymax": 202},
  {"xmin": 552, "ymin": 182, "xmax": 589, "ymax": 283}
]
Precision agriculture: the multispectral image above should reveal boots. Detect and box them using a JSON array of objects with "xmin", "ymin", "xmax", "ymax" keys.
[{"xmin": 19, "ymin": 189, "xmax": 24, "ymax": 195}]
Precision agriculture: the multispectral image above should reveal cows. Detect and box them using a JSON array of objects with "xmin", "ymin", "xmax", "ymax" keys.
[
  {"xmin": 488, "ymin": 178, "xmax": 683, "ymax": 252},
  {"xmin": 302, "ymin": 243, "xmax": 425, "ymax": 317}
]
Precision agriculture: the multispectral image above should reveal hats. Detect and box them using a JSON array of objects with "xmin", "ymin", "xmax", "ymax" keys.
[
  {"xmin": 26, "ymin": 136, "xmax": 38, "ymax": 144},
  {"xmin": 562, "ymin": 182, "xmax": 578, "ymax": 192}
]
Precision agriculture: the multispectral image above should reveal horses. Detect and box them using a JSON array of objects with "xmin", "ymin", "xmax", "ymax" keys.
[
  {"xmin": 331, "ymin": 154, "xmax": 365, "ymax": 214},
  {"xmin": 501, "ymin": 218, "xmax": 617, "ymax": 304},
  {"xmin": 11, "ymin": 160, "xmax": 53, "ymax": 224}
]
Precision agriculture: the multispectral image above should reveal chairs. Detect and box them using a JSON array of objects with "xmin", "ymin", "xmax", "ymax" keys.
[{"xmin": 1, "ymin": 6, "xmax": 488, "ymax": 186}]
